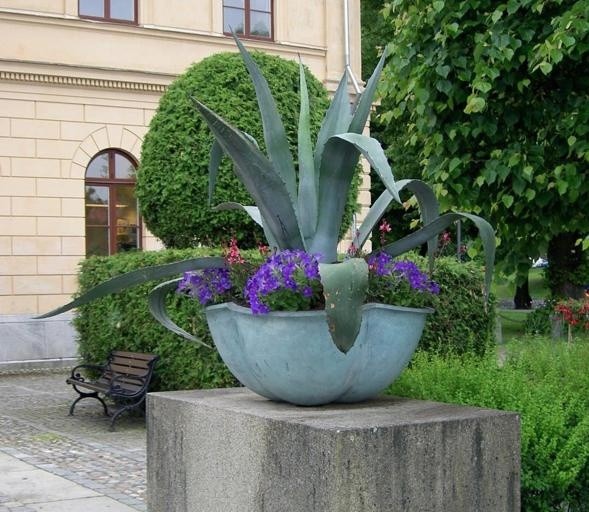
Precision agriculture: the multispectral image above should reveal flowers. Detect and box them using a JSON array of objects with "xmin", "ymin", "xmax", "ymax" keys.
[{"xmin": 29, "ymin": 24, "xmax": 496, "ymax": 355}]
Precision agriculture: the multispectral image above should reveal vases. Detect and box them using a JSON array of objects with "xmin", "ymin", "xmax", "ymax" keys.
[{"xmin": 201, "ymin": 303, "xmax": 437, "ymax": 406}]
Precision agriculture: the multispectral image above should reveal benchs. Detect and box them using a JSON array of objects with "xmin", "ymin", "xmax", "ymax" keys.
[{"xmin": 65, "ymin": 349, "xmax": 160, "ymax": 431}]
